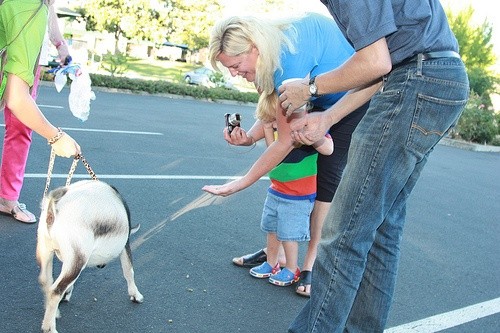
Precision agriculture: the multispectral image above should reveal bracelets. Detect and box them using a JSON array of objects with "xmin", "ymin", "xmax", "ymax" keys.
[{"xmin": 48, "ymin": 127, "xmax": 64, "ymax": 145}]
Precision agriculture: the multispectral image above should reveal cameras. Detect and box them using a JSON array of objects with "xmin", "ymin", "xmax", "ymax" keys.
[{"xmin": 224, "ymin": 113, "xmax": 241, "ymax": 134}]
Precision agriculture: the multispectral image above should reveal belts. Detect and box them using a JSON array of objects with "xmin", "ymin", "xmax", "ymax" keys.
[{"xmin": 388, "ymin": 50, "xmax": 460, "ymax": 75}]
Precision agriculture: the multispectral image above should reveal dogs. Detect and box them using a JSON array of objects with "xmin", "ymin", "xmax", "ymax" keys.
[{"xmin": 37, "ymin": 181, "xmax": 146, "ymax": 332}]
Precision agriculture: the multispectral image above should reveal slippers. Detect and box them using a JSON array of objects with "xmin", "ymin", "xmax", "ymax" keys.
[
  {"xmin": 233, "ymin": 249, "xmax": 267, "ymax": 268},
  {"xmin": 0, "ymin": 203, "xmax": 37, "ymax": 224},
  {"xmin": 296, "ymin": 270, "xmax": 311, "ymax": 297}
]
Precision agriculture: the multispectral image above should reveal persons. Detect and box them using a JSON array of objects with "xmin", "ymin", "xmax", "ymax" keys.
[
  {"xmin": 0, "ymin": 0, "xmax": 83, "ymax": 226},
  {"xmin": 278, "ymin": 0, "xmax": 469, "ymax": 333},
  {"xmin": 249, "ymin": 88, "xmax": 334, "ymax": 287},
  {"xmin": 201, "ymin": 13, "xmax": 375, "ymax": 295}
]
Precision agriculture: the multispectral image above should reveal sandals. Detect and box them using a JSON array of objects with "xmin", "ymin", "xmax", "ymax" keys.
[
  {"xmin": 269, "ymin": 267, "xmax": 301, "ymax": 287},
  {"xmin": 249, "ymin": 261, "xmax": 281, "ymax": 278}
]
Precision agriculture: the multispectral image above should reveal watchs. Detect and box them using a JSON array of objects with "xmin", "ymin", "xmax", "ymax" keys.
[{"xmin": 308, "ymin": 75, "xmax": 320, "ymax": 97}]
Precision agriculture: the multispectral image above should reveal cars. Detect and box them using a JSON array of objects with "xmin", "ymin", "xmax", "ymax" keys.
[{"xmin": 183, "ymin": 68, "xmax": 233, "ymax": 89}]
